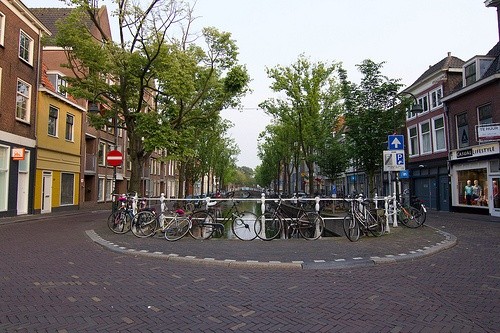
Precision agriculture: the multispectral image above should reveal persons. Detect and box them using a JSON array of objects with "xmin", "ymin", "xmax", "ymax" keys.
[
  {"xmin": 493, "ymin": 181, "xmax": 499, "ymax": 208},
  {"xmin": 473, "ymin": 180, "xmax": 481, "ymax": 205},
  {"xmin": 464, "ymin": 180, "xmax": 473, "ymax": 205},
  {"xmin": 483, "ymin": 181, "xmax": 488, "ymax": 204}
]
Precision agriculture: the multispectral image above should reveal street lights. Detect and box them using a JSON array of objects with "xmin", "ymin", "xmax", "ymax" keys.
[{"xmin": 393, "ymin": 91, "xmax": 423, "ymax": 135}]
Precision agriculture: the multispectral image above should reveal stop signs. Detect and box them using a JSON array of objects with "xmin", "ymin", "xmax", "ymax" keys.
[{"xmin": 107, "ymin": 149, "xmax": 124, "ymax": 167}]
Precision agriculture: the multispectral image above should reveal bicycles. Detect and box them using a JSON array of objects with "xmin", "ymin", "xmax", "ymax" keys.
[
  {"xmin": 130, "ymin": 207, "xmax": 193, "ymax": 242},
  {"xmin": 392, "ymin": 189, "xmax": 431, "ymax": 228},
  {"xmin": 187, "ymin": 201, "xmax": 262, "ymax": 242},
  {"xmin": 253, "ymin": 197, "xmax": 326, "ymax": 242},
  {"xmin": 107, "ymin": 197, "xmax": 158, "ymax": 237},
  {"xmin": 343, "ymin": 197, "xmax": 384, "ymax": 243}
]
